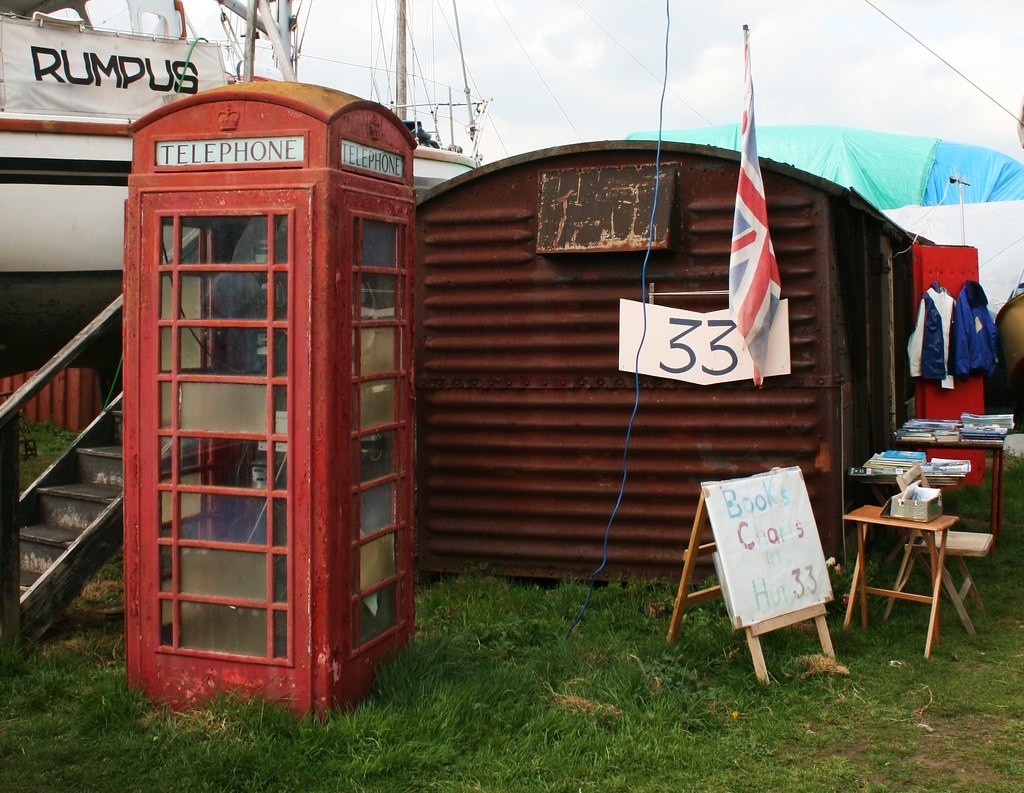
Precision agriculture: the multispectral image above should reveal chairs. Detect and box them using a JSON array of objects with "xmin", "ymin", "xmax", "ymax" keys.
[{"xmin": 885, "ymin": 459, "xmax": 993, "ymax": 636}]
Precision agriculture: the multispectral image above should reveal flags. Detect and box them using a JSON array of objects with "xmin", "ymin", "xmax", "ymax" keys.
[{"xmin": 729, "ymin": 39, "xmax": 783, "ymax": 390}]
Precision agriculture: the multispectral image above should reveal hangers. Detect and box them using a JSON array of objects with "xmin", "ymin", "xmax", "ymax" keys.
[{"xmin": 932, "ymin": 271, "xmax": 941, "ymax": 288}]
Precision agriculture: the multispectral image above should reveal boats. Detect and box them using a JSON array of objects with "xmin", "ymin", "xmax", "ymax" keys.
[{"xmin": 0, "ymin": 0, "xmax": 487, "ymax": 372}]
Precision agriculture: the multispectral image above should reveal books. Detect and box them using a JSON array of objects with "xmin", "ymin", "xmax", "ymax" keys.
[{"xmin": 864, "ymin": 408, "xmax": 1015, "ymax": 487}]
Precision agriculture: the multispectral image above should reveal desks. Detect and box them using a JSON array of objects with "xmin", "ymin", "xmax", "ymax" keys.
[
  {"xmin": 895, "ymin": 438, "xmax": 1004, "ymax": 555},
  {"xmin": 858, "ymin": 475, "xmax": 960, "ymax": 579},
  {"xmin": 842, "ymin": 503, "xmax": 960, "ymax": 660}
]
